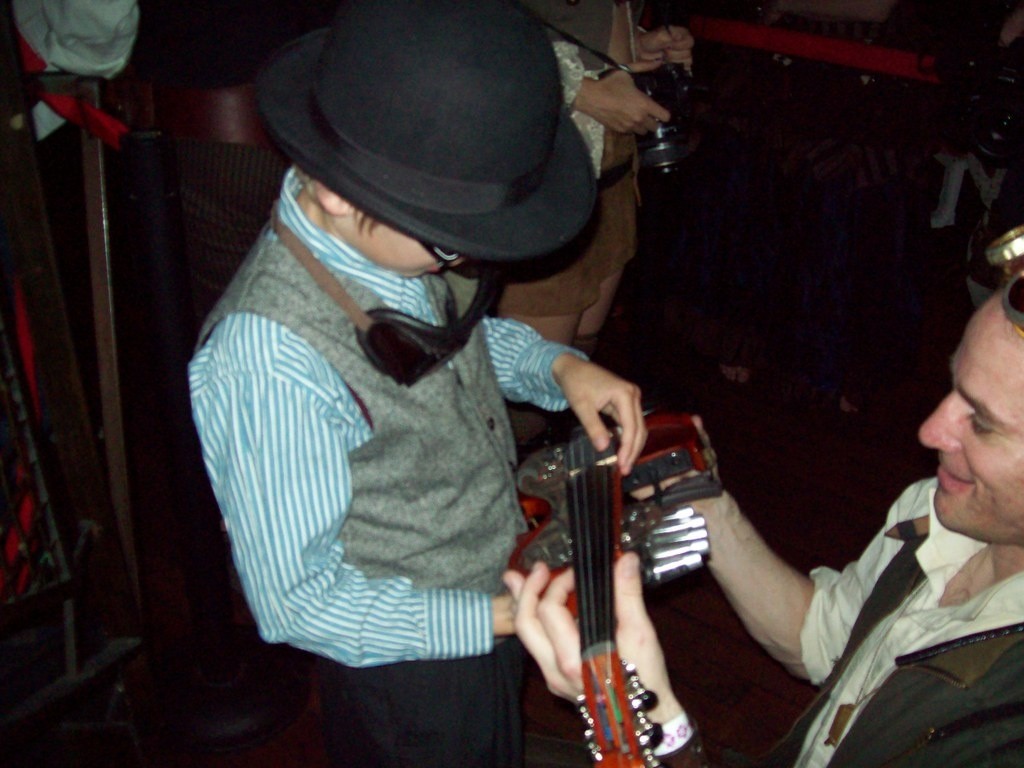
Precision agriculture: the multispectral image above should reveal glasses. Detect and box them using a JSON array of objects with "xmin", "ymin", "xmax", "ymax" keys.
[
  {"xmin": 354, "ymin": 263, "xmax": 501, "ymax": 387},
  {"xmin": 985, "ymin": 223, "xmax": 1024, "ymax": 344}
]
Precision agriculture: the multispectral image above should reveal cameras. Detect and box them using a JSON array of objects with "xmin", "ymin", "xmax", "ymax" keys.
[{"xmin": 604, "ymin": 60, "xmax": 694, "ymax": 174}]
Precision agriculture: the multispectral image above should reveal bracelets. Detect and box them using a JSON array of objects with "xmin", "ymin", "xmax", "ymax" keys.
[{"xmin": 649, "ymin": 712, "xmax": 694, "ymax": 757}]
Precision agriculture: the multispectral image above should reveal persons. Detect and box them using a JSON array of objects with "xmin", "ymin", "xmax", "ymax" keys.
[{"xmin": 153, "ymin": 0, "xmax": 1024, "ymax": 768}]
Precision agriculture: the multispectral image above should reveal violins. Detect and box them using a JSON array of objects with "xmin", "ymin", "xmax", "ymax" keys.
[{"xmin": 505, "ymin": 412, "xmax": 712, "ymax": 768}]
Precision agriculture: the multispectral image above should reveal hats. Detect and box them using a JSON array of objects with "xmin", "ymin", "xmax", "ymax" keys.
[{"xmin": 253, "ymin": 0, "xmax": 595, "ymax": 259}]
